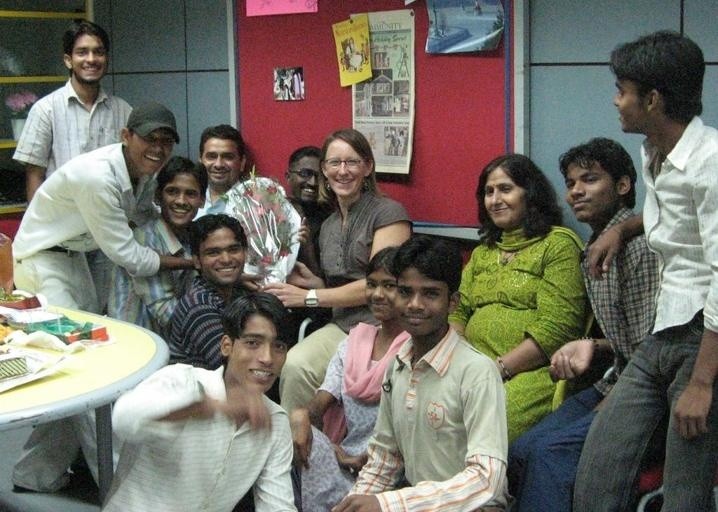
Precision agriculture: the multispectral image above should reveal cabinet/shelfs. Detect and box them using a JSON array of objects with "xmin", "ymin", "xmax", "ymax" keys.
[{"xmin": 0, "ymin": 0, "xmax": 98, "ymax": 213}]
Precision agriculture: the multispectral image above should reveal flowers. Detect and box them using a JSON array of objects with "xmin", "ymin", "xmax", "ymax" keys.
[{"xmin": 3, "ymin": 89, "xmax": 40, "ymax": 117}]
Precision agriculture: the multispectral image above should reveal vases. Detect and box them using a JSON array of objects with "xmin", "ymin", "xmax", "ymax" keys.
[{"xmin": 12, "ymin": 116, "xmax": 28, "ymax": 138}]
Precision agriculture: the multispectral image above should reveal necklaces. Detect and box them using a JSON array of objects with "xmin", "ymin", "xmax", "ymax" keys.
[{"xmin": 501, "ymin": 250, "xmax": 516, "ymax": 264}]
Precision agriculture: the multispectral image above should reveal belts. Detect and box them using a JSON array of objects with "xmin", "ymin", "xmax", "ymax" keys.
[{"xmin": 44, "ymin": 245, "xmax": 74, "ymax": 258}]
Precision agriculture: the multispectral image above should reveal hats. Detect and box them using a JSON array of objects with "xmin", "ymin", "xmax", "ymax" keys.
[{"xmin": 127, "ymin": 103, "xmax": 180, "ymax": 145}]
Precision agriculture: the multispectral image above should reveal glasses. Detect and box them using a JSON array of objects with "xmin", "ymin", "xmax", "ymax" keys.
[
  {"xmin": 324, "ymin": 158, "xmax": 369, "ymax": 166},
  {"xmin": 142, "ymin": 133, "xmax": 176, "ymax": 146},
  {"xmin": 288, "ymin": 168, "xmax": 319, "ymax": 178}
]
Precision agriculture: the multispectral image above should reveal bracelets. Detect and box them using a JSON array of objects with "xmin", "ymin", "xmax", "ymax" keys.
[
  {"xmin": 578, "ymin": 336, "xmax": 599, "ymax": 360},
  {"xmin": 497, "ymin": 356, "xmax": 511, "ymax": 380},
  {"xmin": 304, "ymin": 289, "xmax": 320, "ymax": 309}
]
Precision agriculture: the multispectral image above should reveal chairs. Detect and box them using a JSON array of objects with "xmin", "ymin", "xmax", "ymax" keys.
[{"xmin": 629, "ymin": 434, "xmax": 717, "ymax": 512}]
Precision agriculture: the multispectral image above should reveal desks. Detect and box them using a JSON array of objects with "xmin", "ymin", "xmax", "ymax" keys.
[{"xmin": 0, "ymin": 299, "xmax": 167, "ymax": 502}]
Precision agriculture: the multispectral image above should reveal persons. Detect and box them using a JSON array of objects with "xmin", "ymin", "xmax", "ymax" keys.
[
  {"xmin": 12, "ymin": 101, "xmax": 200, "ymax": 495},
  {"xmin": 446, "ymin": 153, "xmax": 592, "ymax": 450},
  {"xmin": 324, "ymin": 234, "xmax": 517, "ymax": 511},
  {"xmin": 572, "ymin": 29, "xmax": 717, "ymax": 510},
  {"xmin": 277, "ymin": 147, "xmax": 332, "ymax": 349},
  {"xmin": 96, "ymin": 292, "xmax": 299, "ymax": 510},
  {"xmin": 506, "ymin": 138, "xmax": 627, "ymax": 511},
  {"xmin": 160, "ymin": 213, "xmax": 282, "ymax": 404},
  {"xmin": 106, "ymin": 153, "xmax": 263, "ymax": 344},
  {"xmin": 189, "ymin": 121, "xmax": 303, "ymax": 294},
  {"xmin": 265, "ymin": 127, "xmax": 416, "ymax": 414},
  {"xmin": 10, "ymin": 18, "xmax": 135, "ymax": 208},
  {"xmin": 283, "ymin": 245, "xmax": 412, "ymax": 510}
]
